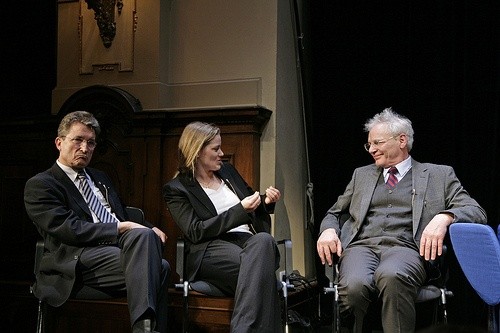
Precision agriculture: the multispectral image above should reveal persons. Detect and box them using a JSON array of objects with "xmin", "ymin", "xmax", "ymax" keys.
[
  {"xmin": 316, "ymin": 108, "xmax": 487, "ymax": 333},
  {"xmin": 162, "ymin": 121, "xmax": 284, "ymax": 333},
  {"xmin": 25, "ymin": 111, "xmax": 170, "ymax": 333}
]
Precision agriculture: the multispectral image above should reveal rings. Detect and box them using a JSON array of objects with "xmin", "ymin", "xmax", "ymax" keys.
[{"xmin": 257, "ymin": 204, "xmax": 259, "ymax": 206}]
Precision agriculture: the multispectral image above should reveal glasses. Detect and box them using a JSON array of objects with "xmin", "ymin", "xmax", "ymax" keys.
[
  {"xmin": 66, "ymin": 137, "xmax": 96, "ymax": 148},
  {"xmin": 364, "ymin": 136, "xmax": 394, "ymax": 151}
]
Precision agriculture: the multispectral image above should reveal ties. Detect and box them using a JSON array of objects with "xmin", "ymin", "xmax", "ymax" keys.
[
  {"xmin": 385, "ymin": 167, "xmax": 398, "ymax": 187},
  {"xmin": 78, "ymin": 169, "xmax": 118, "ymax": 223}
]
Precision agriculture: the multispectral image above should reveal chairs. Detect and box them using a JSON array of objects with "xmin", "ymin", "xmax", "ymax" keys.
[
  {"xmin": 31, "ymin": 205, "xmax": 170, "ymax": 333},
  {"xmin": 324, "ymin": 222, "xmax": 500, "ymax": 333},
  {"xmin": 175, "ymin": 234, "xmax": 293, "ymax": 333}
]
